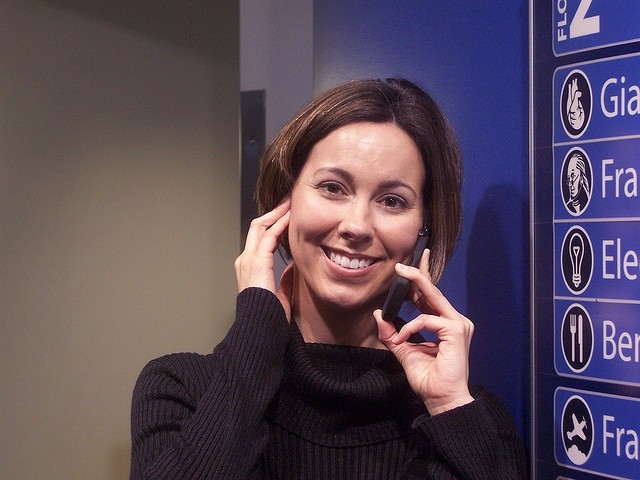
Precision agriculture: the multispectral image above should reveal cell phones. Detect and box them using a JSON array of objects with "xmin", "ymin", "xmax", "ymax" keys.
[{"xmin": 382, "ymin": 209, "xmax": 430, "ymax": 323}]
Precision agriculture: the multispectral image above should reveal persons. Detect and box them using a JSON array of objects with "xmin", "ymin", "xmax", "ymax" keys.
[{"xmin": 128, "ymin": 78, "xmax": 529, "ymax": 479}]
